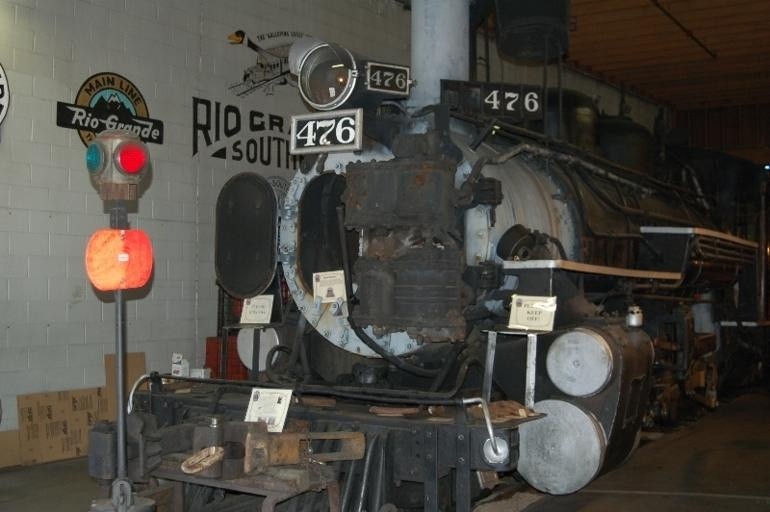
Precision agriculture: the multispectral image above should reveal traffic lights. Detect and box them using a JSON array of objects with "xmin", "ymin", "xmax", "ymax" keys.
[
  {"xmin": 84, "ymin": 139, "xmax": 111, "ymax": 184},
  {"xmin": 113, "ymin": 139, "xmax": 149, "ymax": 183}
]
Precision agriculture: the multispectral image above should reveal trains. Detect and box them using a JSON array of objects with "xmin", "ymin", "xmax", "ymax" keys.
[{"xmin": 122, "ymin": 37, "xmax": 769, "ymax": 511}]
